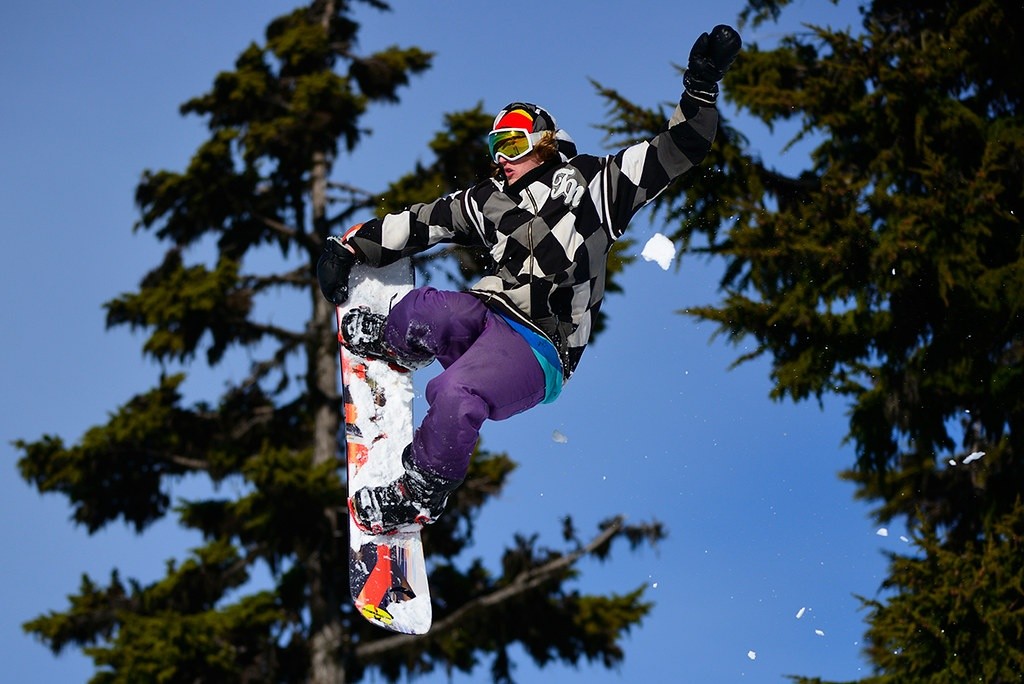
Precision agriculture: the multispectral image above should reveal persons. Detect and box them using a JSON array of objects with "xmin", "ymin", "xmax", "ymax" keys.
[{"xmin": 317, "ymin": 23, "xmax": 742, "ymax": 534}]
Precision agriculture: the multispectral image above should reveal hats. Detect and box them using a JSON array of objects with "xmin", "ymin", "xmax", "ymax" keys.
[{"xmin": 494, "ymin": 108, "xmax": 534, "ymax": 132}]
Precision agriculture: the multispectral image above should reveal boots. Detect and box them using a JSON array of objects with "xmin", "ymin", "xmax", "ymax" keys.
[
  {"xmin": 353, "ymin": 446, "xmax": 463, "ymax": 531},
  {"xmin": 340, "ymin": 310, "xmax": 433, "ymax": 372}
]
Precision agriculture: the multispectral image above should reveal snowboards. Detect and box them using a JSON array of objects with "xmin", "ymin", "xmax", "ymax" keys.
[{"xmin": 336, "ymin": 223, "xmax": 432, "ymax": 634}]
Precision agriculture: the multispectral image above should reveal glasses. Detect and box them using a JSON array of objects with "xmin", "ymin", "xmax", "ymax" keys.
[{"xmin": 488, "ymin": 128, "xmax": 534, "ymax": 165}]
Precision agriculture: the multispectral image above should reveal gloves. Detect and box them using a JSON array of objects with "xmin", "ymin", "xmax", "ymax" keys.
[
  {"xmin": 318, "ymin": 235, "xmax": 355, "ymax": 307},
  {"xmin": 685, "ymin": 24, "xmax": 742, "ymax": 96}
]
